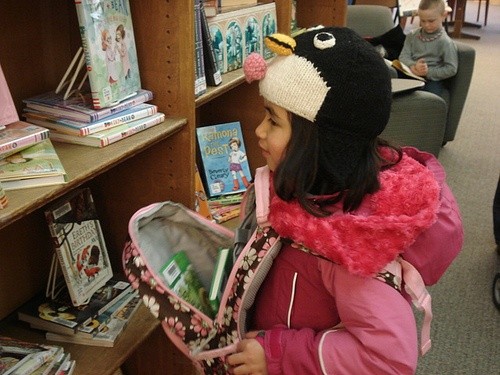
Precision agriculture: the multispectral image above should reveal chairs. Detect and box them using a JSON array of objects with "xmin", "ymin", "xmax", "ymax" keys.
[
  {"xmin": 347, "ymin": 5, "xmax": 392, "ymax": 38},
  {"xmin": 378, "ymin": 90, "xmax": 447, "ymax": 161},
  {"xmin": 438, "ymin": 43, "xmax": 476, "ymax": 147}
]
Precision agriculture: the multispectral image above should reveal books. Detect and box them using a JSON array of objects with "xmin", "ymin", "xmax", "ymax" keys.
[
  {"xmin": 157, "ymin": 248, "xmax": 234, "ymax": 321},
  {"xmin": 0, "ymin": 66, "xmax": 69, "ymax": 185},
  {"xmin": 192, "ymin": 1, "xmax": 223, "ymax": 99},
  {"xmin": 194, "ymin": 120, "xmax": 253, "ymax": 226},
  {"xmin": 21, "ymin": 0, "xmax": 165, "ymax": 148},
  {"xmin": 0, "ymin": 335, "xmax": 76, "ymax": 375},
  {"xmin": 18, "ymin": 186, "xmax": 142, "ymax": 348}
]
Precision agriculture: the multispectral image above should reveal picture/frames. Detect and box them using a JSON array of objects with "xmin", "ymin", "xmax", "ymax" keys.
[{"xmin": 45, "ymin": 186, "xmax": 114, "ymax": 307}]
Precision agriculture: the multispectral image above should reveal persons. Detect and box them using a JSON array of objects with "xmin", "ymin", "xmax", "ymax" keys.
[
  {"xmin": 399, "ymin": 0, "xmax": 458, "ymax": 105},
  {"xmin": 225, "ymin": 26, "xmax": 441, "ymax": 374},
  {"xmin": 351, "ymin": 0, "xmax": 406, "ymax": 60}
]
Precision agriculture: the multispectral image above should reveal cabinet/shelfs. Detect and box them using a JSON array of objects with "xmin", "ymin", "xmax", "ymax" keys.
[{"xmin": 0, "ymin": 0, "xmax": 346, "ymax": 375}]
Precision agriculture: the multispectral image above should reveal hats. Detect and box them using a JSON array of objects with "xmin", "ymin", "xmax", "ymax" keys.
[{"xmin": 244, "ymin": 26, "xmax": 393, "ymax": 138}]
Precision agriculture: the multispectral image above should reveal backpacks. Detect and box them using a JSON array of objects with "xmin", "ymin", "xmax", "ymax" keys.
[{"xmin": 122, "ymin": 165, "xmax": 432, "ymax": 375}]
